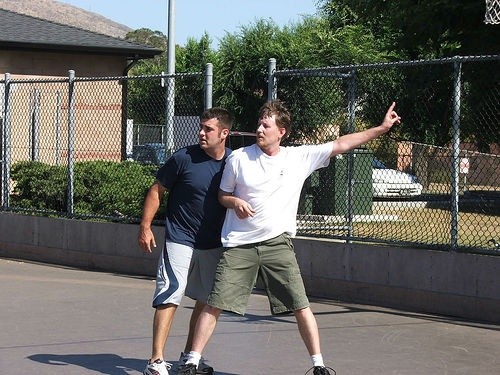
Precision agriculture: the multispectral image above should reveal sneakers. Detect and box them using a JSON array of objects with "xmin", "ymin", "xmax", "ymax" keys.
[
  {"xmin": 313, "ymin": 366, "xmax": 331, "ymax": 375},
  {"xmin": 143, "ymin": 359, "xmax": 173, "ymax": 375},
  {"xmin": 177, "ymin": 363, "xmax": 197, "ymax": 375},
  {"xmin": 176, "ymin": 352, "xmax": 214, "ymax": 375}
]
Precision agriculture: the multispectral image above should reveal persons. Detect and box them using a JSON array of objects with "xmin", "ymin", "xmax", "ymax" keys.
[
  {"xmin": 184, "ymin": 99, "xmax": 401, "ymax": 375},
  {"xmin": 139, "ymin": 108, "xmax": 236, "ymax": 375}
]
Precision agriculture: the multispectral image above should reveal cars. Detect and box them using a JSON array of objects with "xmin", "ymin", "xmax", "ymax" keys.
[
  {"xmin": 371, "ymin": 158, "xmax": 423, "ymax": 199},
  {"xmin": 132, "ymin": 143, "xmax": 167, "ymax": 166}
]
